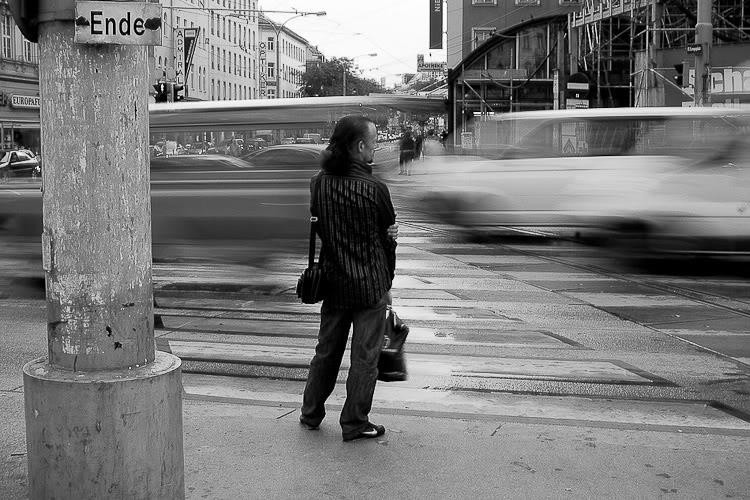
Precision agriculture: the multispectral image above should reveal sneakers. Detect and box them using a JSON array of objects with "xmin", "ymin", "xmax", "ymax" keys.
[
  {"xmin": 300, "ymin": 415, "xmax": 319, "ymax": 430},
  {"xmin": 344, "ymin": 422, "xmax": 385, "ymax": 441}
]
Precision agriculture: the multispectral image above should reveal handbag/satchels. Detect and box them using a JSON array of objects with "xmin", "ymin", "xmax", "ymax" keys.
[
  {"xmin": 376, "ymin": 310, "xmax": 409, "ymax": 381},
  {"xmin": 297, "ymin": 262, "xmax": 327, "ymax": 303}
]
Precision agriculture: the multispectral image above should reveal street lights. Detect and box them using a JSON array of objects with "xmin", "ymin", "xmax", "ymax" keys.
[
  {"xmin": 227, "ymin": 10, "xmax": 327, "ymax": 98},
  {"xmin": 342, "ymin": 53, "xmax": 378, "ymax": 96}
]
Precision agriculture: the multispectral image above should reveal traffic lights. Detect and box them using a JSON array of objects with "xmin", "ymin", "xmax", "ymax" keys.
[
  {"xmin": 173, "ymin": 84, "xmax": 184, "ymax": 101},
  {"xmin": 153, "ymin": 83, "xmax": 166, "ymax": 102}
]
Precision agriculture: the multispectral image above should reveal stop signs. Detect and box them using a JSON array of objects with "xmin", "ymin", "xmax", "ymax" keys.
[{"xmin": 566, "ymin": 73, "xmax": 590, "ymax": 98}]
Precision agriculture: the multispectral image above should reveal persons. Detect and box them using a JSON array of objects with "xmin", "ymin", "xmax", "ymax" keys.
[
  {"xmin": 298, "ymin": 113, "xmax": 400, "ymax": 442},
  {"xmin": 398, "ymin": 126, "xmax": 440, "ymax": 177}
]
